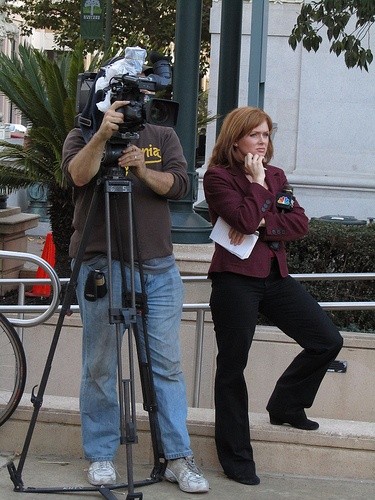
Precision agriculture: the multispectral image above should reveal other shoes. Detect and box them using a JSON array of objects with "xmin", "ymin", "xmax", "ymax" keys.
[
  {"xmin": 269, "ymin": 411, "xmax": 319, "ymax": 430},
  {"xmin": 227, "ymin": 475, "xmax": 260, "ymax": 485}
]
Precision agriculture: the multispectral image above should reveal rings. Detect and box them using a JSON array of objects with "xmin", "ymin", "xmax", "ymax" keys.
[{"xmin": 134, "ymin": 155, "xmax": 137, "ymax": 160}]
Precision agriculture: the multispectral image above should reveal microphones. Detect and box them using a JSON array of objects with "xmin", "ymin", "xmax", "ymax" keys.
[{"xmin": 272, "ymin": 185, "xmax": 294, "ymax": 250}]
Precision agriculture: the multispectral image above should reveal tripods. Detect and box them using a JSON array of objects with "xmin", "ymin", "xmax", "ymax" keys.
[{"xmin": 7, "ymin": 133, "xmax": 167, "ymax": 500}]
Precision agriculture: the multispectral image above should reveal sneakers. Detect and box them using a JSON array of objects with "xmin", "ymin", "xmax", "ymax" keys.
[
  {"xmin": 164, "ymin": 456, "xmax": 209, "ymax": 493},
  {"xmin": 87, "ymin": 460, "xmax": 116, "ymax": 486}
]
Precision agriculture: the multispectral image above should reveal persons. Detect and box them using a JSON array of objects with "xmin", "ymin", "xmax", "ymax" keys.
[
  {"xmin": 60, "ymin": 60, "xmax": 210, "ymax": 496},
  {"xmin": 199, "ymin": 106, "xmax": 344, "ymax": 485}
]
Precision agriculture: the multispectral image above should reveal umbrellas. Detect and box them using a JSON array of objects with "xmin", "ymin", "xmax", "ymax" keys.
[{"xmin": 32, "ymin": 233, "xmax": 56, "ymax": 301}]
